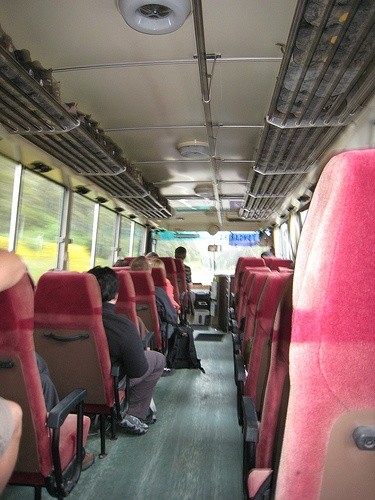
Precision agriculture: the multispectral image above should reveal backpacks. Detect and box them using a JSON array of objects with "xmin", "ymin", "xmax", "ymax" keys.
[{"xmin": 166, "ymin": 326, "xmax": 206, "ymax": 373}]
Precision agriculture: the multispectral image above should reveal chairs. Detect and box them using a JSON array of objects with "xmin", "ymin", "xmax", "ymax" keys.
[
  {"xmin": 151, "ymin": 268, "xmax": 181, "ymax": 338},
  {"xmin": 0, "ymin": 274, "xmax": 87, "ymax": 500},
  {"xmin": 130, "ymin": 271, "xmax": 169, "ymax": 357},
  {"xmin": 115, "ymin": 270, "xmax": 156, "ymax": 351},
  {"xmin": 160, "ymin": 256, "xmax": 191, "ymax": 325},
  {"xmin": 34, "ymin": 271, "xmax": 130, "ymax": 459},
  {"xmin": 227, "ymin": 149, "xmax": 375, "ymax": 500}
]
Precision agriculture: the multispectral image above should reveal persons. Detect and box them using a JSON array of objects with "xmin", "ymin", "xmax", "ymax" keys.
[
  {"xmin": 112, "ymin": 252, "xmax": 181, "ymax": 326},
  {"xmin": 86, "ymin": 264, "xmax": 165, "ymax": 434},
  {"xmin": 34, "ymin": 353, "xmax": 95, "ymax": 473},
  {"xmin": 175, "ymin": 246, "xmax": 196, "ymax": 314},
  {"xmin": 261, "ymin": 251, "xmax": 274, "ymax": 259},
  {"xmin": 0, "ymin": 396, "xmax": 24, "ymax": 495}
]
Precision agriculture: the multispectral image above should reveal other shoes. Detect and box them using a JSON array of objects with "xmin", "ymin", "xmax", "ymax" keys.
[{"xmin": 160, "ymin": 368, "xmax": 176, "ymax": 377}]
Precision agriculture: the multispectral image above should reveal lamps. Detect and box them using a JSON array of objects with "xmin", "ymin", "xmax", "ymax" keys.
[
  {"xmin": 176, "ymin": 141, "xmax": 210, "ymax": 158},
  {"xmin": 194, "ymin": 188, "xmax": 213, "ymax": 198},
  {"xmin": 115, "ymin": 0, "xmax": 193, "ymax": 35}
]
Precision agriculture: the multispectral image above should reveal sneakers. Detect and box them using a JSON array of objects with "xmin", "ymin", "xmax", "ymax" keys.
[{"xmin": 120, "ymin": 414, "xmax": 148, "ymax": 434}]
